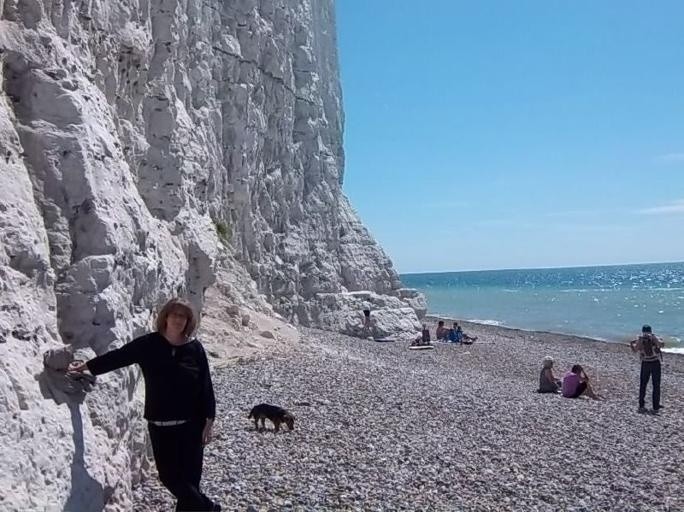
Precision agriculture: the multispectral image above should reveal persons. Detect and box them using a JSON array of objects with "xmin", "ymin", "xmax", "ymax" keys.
[
  {"xmin": 362, "ymin": 298, "xmax": 376, "ymax": 330},
  {"xmin": 436, "ymin": 320, "xmax": 449, "ymax": 339},
  {"xmin": 536, "ymin": 355, "xmax": 562, "ymax": 394},
  {"xmin": 630, "ymin": 324, "xmax": 667, "ymax": 411},
  {"xmin": 457, "ymin": 326, "xmax": 478, "ymax": 341},
  {"xmin": 68, "ymin": 298, "xmax": 223, "ymax": 512},
  {"xmin": 449, "ymin": 322, "xmax": 458, "ymax": 341},
  {"xmin": 410, "ymin": 322, "xmax": 431, "ymax": 347},
  {"xmin": 562, "ymin": 363, "xmax": 600, "ymax": 399}
]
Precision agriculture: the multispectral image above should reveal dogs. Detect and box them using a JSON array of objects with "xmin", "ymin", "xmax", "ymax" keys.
[{"xmin": 247, "ymin": 403, "xmax": 294, "ymax": 431}]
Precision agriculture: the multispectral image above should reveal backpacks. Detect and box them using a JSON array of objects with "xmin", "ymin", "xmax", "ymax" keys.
[{"xmin": 636, "ymin": 334, "xmax": 663, "ymax": 363}]
